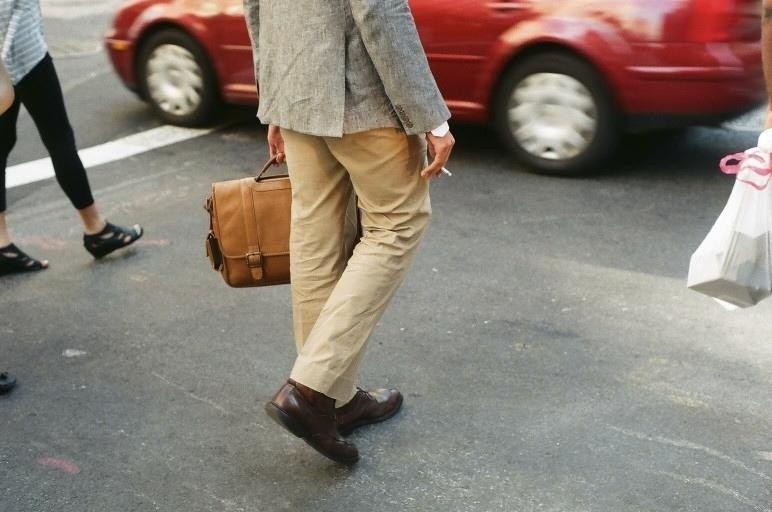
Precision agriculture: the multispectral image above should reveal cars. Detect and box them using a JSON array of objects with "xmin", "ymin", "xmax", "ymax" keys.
[{"xmin": 107, "ymin": 0, "xmax": 769, "ymax": 177}]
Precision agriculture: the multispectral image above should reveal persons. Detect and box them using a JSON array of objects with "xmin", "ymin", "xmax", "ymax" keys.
[
  {"xmin": 243, "ymin": 0, "xmax": 456, "ymax": 464},
  {"xmin": 0, "ymin": 0, "xmax": 144, "ymax": 278},
  {"xmin": 760, "ymin": 0, "xmax": 772, "ymax": 129}
]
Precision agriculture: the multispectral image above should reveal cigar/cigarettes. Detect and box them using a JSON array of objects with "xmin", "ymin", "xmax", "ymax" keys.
[{"xmin": 441, "ymin": 166, "xmax": 452, "ymax": 177}]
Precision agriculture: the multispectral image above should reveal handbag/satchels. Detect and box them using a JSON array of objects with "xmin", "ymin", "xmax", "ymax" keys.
[{"xmin": 203, "ymin": 155, "xmax": 362, "ymax": 288}]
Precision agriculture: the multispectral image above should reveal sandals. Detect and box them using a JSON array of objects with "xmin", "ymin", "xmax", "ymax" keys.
[
  {"xmin": 84, "ymin": 223, "xmax": 144, "ymax": 259},
  {"xmin": 0, "ymin": 242, "xmax": 49, "ymax": 276}
]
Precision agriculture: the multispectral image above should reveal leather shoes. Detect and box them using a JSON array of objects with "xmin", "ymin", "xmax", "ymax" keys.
[{"xmin": 265, "ymin": 382, "xmax": 403, "ymax": 464}]
